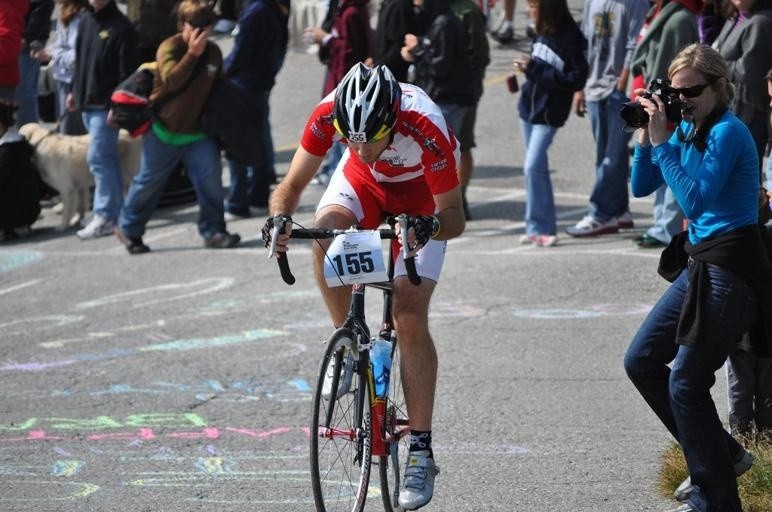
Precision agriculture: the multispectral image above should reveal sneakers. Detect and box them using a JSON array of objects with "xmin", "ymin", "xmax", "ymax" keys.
[
  {"xmin": 520, "ymin": 235, "xmax": 558, "ymax": 246},
  {"xmin": 77, "ymin": 214, "xmax": 115, "ymax": 240},
  {"xmin": 115, "ymin": 227, "xmax": 150, "ymax": 253},
  {"xmin": 617, "ymin": 210, "xmax": 634, "ymax": 229},
  {"xmin": 206, "ymin": 232, "xmax": 241, "ymax": 248},
  {"xmin": 250, "ymin": 189, "xmax": 271, "ymax": 207},
  {"xmin": 526, "ymin": 23, "xmax": 537, "ymax": 43},
  {"xmin": 492, "ymin": 20, "xmax": 514, "ymax": 42},
  {"xmin": 398, "ymin": 450, "xmax": 437, "ymax": 510},
  {"xmin": 228, "ymin": 205, "xmax": 250, "ymax": 218},
  {"xmin": 673, "ymin": 449, "xmax": 754, "ymax": 501},
  {"xmin": 565, "ymin": 214, "xmax": 619, "ymax": 236},
  {"xmin": 668, "ymin": 485, "xmax": 707, "ymax": 511},
  {"xmin": 322, "ymin": 346, "xmax": 356, "ymax": 399}
]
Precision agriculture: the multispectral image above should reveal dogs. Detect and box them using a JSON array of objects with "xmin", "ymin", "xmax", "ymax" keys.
[{"xmin": 17, "ymin": 122, "xmax": 144, "ymax": 232}]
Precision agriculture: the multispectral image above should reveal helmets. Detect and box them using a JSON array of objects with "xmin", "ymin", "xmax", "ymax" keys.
[{"xmin": 334, "ymin": 62, "xmax": 401, "ymax": 143}]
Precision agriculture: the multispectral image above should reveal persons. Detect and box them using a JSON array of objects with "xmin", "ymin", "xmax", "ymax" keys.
[
  {"xmin": 258, "ymin": 61, "xmax": 469, "ymax": 509},
  {"xmin": 0, "ymin": 1, "xmax": 292, "ymax": 254},
  {"xmin": 302, "ymin": 1, "xmax": 491, "ymax": 219},
  {"xmin": 566, "ymin": 0, "xmax": 771, "ymax": 247},
  {"xmin": 513, "ymin": 1, "xmax": 589, "ymax": 248},
  {"xmin": 495, "ymin": 0, "xmax": 537, "ymax": 45},
  {"xmin": 618, "ymin": 41, "xmax": 764, "ymax": 510}
]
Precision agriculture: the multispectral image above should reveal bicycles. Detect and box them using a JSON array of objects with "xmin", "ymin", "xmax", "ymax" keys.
[{"xmin": 268, "ymin": 211, "xmax": 422, "ymax": 512}]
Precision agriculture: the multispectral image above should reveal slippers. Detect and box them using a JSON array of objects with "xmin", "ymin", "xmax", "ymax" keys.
[
  {"xmin": 631, "ymin": 233, "xmax": 652, "ymax": 243},
  {"xmin": 637, "ymin": 238, "xmax": 663, "ymax": 247}
]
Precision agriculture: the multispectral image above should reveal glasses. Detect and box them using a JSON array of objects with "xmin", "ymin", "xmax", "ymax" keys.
[
  {"xmin": 669, "ymin": 76, "xmax": 729, "ymax": 98},
  {"xmin": 184, "ymin": 20, "xmax": 217, "ymax": 27}
]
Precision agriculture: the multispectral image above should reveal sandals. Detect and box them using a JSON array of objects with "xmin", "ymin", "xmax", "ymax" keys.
[{"xmin": 310, "ymin": 168, "xmax": 333, "ymax": 186}]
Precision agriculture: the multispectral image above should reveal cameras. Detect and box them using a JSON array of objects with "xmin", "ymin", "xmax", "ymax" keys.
[{"xmin": 621, "ymin": 79, "xmax": 682, "ymax": 127}]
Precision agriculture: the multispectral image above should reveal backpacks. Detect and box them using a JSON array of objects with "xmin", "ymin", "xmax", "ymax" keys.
[{"xmin": 111, "ymin": 62, "xmax": 202, "ymax": 139}]
